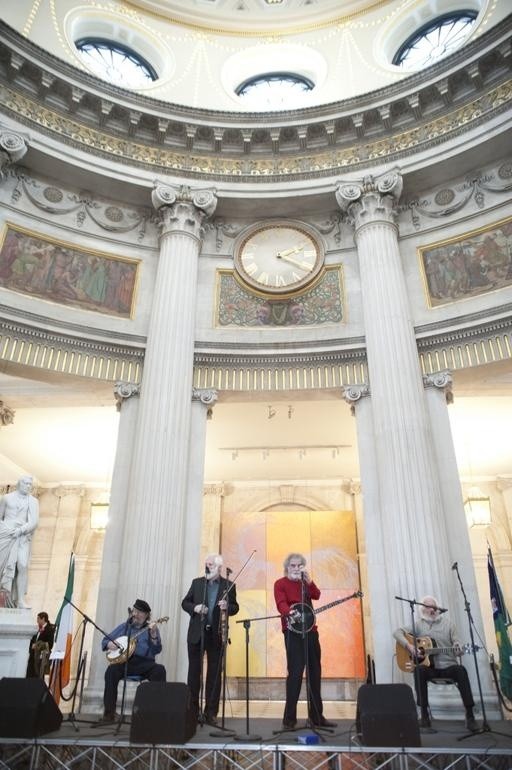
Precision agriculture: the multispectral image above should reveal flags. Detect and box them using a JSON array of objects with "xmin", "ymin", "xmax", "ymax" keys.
[
  {"xmin": 486, "ymin": 547, "xmax": 512, "ymax": 703},
  {"xmin": 48, "ymin": 555, "xmax": 80, "ymax": 704}
]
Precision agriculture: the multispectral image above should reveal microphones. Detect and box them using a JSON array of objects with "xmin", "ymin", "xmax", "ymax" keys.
[
  {"xmin": 205, "ymin": 566, "xmax": 211, "ymax": 574},
  {"xmin": 119, "ymin": 648, "xmax": 124, "ymax": 654},
  {"xmin": 128, "ymin": 607, "xmax": 137, "ymax": 625},
  {"xmin": 452, "ymin": 562, "xmax": 457, "ymax": 570},
  {"xmin": 439, "ymin": 609, "xmax": 448, "ymax": 614},
  {"xmin": 301, "ymin": 571, "xmax": 306, "ymax": 584}
]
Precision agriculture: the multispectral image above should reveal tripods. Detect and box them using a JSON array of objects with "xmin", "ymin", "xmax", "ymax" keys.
[
  {"xmin": 455, "ymin": 567, "xmax": 512, "ymax": 741},
  {"xmin": 272, "ymin": 581, "xmax": 334, "ymax": 742},
  {"xmin": 197, "ymin": 573, "xmax": 229, "ymax": 731},
  {"xmin": 61, "ymin": 620, "xmax": 100, "ymax": 731},
  {"xmin": 90, "ymin": 618, "xmax": 132, "ymax": 736}
]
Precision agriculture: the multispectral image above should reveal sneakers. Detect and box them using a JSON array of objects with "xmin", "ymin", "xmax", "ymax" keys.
[
  {"xmin": 421, "ymin": 711, "xmax": 431, "ymax": 726},
  {"xmin": 207, "ymin": 714, "xmax": 217, "ymax": 724},
  {"xmin": 315, "ymin": 717, "xmax": 336, "ymax": 727},
  {"xmin": 467, "ymin": 720, "xmax": 479, "ymax": 731},
  {"xmin": 283, "ymin": 721, "xmax": 293, "ymax": 728},
  {"xmin": 102, "ymin": 711, "xmax": 115, "ymax": 721}
]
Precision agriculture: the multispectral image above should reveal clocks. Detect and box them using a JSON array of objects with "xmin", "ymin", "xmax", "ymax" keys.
[{"xmin": 233, "ymin": 217, "xmax": 327, "ymax": 301}]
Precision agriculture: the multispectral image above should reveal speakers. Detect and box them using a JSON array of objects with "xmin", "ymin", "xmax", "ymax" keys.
[
  {"xmin": 356, "ymin": 684, "xmax": 421, "ymax": 747},
  {"xmin": 0, "ymin": 676, "xmax": 64, "ymax": 738},
  {"xmin": 130, "ymin": 682, "xmax": 197, "ymax": 744}
]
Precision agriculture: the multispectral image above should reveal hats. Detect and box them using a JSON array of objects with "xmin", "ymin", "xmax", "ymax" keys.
[{"xmin": 134, "ymin": 599, "xmax": 151, "ymax": 611}]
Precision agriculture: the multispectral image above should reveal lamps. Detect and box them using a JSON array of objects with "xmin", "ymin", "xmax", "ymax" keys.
[
  {"xmin": 465, "ymin": 485, "xmax": 492, "ymax": 530},
  {"xmin": 90, "ymin": 500, "xmax": 111, "ymax": 530}
]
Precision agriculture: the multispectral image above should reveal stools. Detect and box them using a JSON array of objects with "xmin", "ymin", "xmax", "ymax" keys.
[
  {"xmin": 426, "ymin": 678, "xmax": 467, "ymax": 721},
  {"xmin": 118, "ymin": 675, "xmax": 147, "ymax": 683}
]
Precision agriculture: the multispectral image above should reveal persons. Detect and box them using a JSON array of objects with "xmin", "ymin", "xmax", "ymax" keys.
[
  {"xmin": 26, "ymin": 611, "xmax": 54, "ymax": 679},
  {"xmin": 391, "ymin": 594, "xmax": 482, "ymax": 734},
  {"xmin": 0, "ymin": 473, "xmax": 41, "ymax": 612},
  {"xmin": 181, "ymin": 553, "xmax": 240, "ymax": 724},
  {"xmin": 273, "ymin": 554, "xmax": 339, "ymax": 731},
  {"xmin": 94, "ymin": 599, "xmax": 168, "ymax": 720}
]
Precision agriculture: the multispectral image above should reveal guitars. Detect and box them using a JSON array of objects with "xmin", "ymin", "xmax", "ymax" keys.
[{"xmin": 395, "ymin": 633, "xmax": 479, "ymax": 673}]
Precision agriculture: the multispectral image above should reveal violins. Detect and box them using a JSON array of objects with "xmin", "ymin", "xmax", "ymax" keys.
[{"xmin": 217, "ymin": 568, "xmax": 232, "ymax": 646}]
